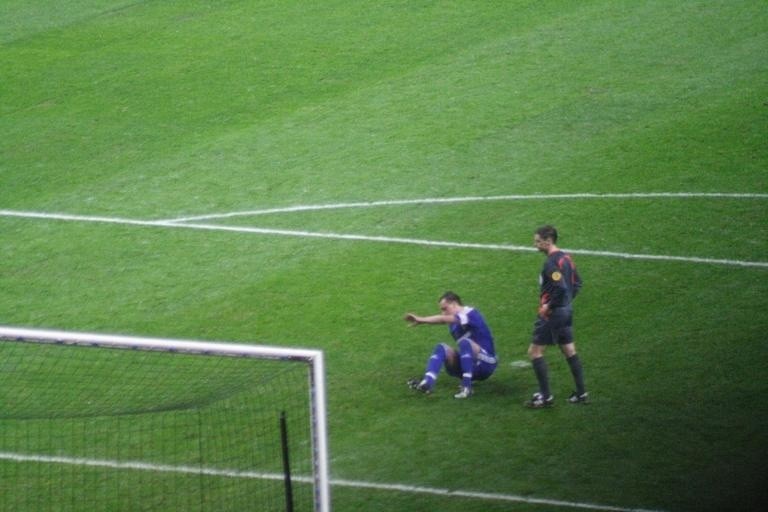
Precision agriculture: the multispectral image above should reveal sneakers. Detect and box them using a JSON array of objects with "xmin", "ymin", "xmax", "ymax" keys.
[
  {"xmin": 524, "ymin": 393, "xmax": 555, "ymax": 409},
  {"xmin": 567, "ymin": 391, "xmax": 589, "ymax": 404},
  {"xmin": 454, "ymin": 386, "xmax": 474, "ymax": 399},
  {"xmin": 408, "ymin": 376, "xmax": 432, "ymax": 394}
]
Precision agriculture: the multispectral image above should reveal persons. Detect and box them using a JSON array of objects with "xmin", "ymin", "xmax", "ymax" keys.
[
  {"xmin": 404, "ymin": 291, "xmax": 496, "ymax": 399},
  {"xmin": 523, "ymin": 225, "xmax": 590, "ymax": 407}
]
